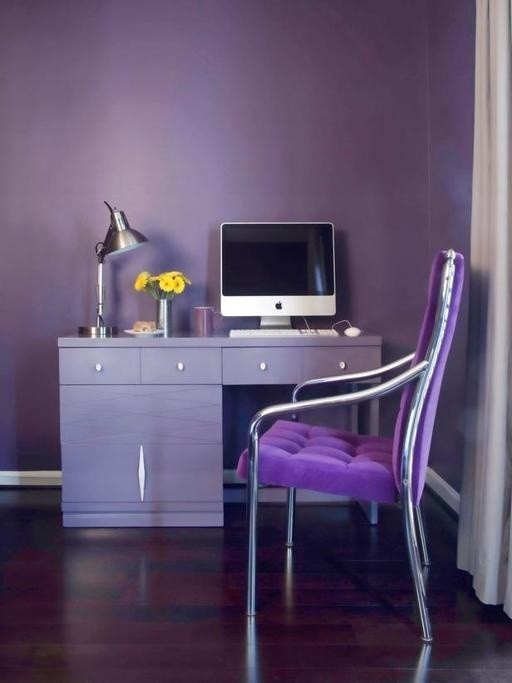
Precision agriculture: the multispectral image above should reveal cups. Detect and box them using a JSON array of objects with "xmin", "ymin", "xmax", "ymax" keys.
[{"xmin": 190, "ymin": 307, "xmax": 214, "ymax": 337}]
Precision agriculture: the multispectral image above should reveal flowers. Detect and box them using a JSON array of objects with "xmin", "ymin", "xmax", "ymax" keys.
[{"xmin": 133, "ymin": 269, "xmax": 192, "ymax": 300}]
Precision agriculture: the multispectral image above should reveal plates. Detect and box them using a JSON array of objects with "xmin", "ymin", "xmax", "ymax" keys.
[{"xmin": 122, "ymin": 329, "xmax": 166, "ymax": 337}]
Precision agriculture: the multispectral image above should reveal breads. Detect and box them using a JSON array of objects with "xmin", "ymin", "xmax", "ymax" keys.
[{"xmin": 133, "ymin": 321, "xmax": 156, "ymax": 332}]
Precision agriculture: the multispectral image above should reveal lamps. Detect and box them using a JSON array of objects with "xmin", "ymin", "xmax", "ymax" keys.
[{"xmin": 78, "ymin": 199, "xmax": 149, "ymax": 337}]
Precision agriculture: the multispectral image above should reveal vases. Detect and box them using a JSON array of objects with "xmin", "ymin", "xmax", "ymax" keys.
[{"xmin": 155, "ymin": 299, "xmax": 172, "ymax": 334}]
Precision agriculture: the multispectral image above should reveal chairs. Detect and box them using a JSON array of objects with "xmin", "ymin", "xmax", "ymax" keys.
[{"xmin": 236, "ymin": 248, "xmax": 466, "ymax": 642}]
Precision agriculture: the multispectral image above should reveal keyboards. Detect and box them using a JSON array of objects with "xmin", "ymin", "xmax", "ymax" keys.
[{"xmin": 229, "ymin": 329, "xmax": 339, "ymax": 338}]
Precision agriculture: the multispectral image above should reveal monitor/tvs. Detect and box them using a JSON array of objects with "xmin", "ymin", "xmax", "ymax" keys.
[{"xmin": 219, "ymin": 221, "xmax": 335, "ymax": 328}]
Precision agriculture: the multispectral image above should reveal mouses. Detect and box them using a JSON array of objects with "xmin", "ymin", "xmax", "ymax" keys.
[{"xmin": 343, "ymin": 326, "xmax": 362, "ymax": 338}]
